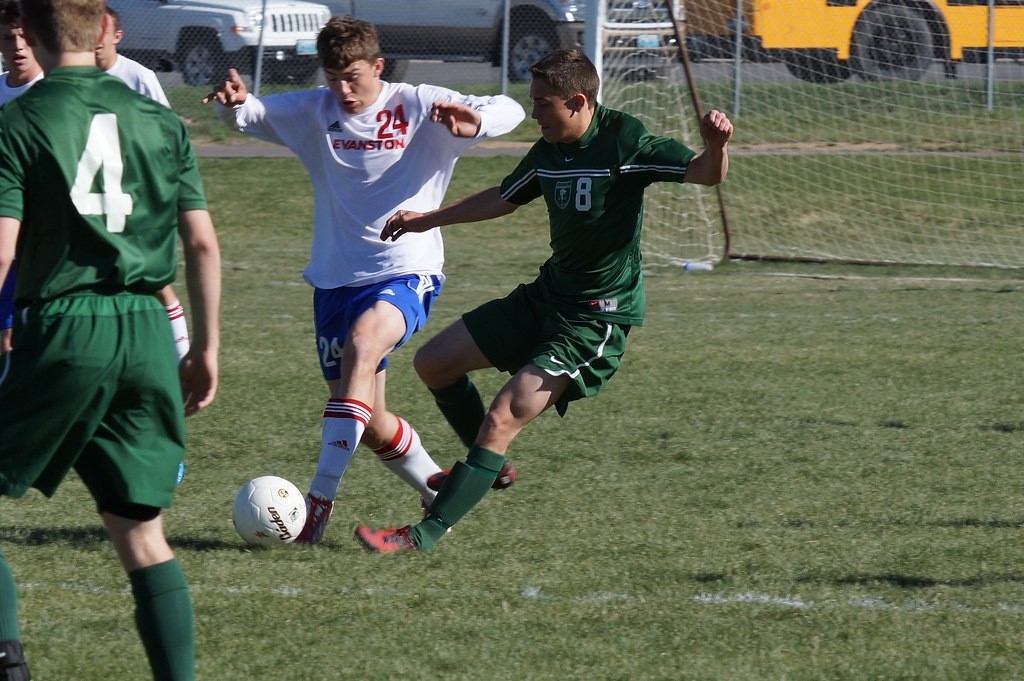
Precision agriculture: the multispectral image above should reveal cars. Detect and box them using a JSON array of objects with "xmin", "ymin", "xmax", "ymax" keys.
[
  {"xmin": 107, "ymin": 0, "xmax": 330, "ymax": 89},
  {"xmin": 309, "ymin": 0, "xmax": 690, "ymax": 86}
]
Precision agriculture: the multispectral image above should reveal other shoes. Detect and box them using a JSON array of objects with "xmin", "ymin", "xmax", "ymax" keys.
[{"xmin": 0, "ymin": 638, "xmax": 33, "ymax": 681}]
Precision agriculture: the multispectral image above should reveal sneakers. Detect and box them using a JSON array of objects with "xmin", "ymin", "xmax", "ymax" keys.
[
  {"xmin": 352, "ymin": 524, "xmax": 416, "ymax": 555},
  {"xmin": 293, "ymin": 489, "xmax": 333, "ymax": 544},
  {"xmin": 425, "ymin": 459, "xmax": 517, "ymax": 490},
  {"xmin": 418, "ymin": 502, "xmax": 451, "ymax": 533}
]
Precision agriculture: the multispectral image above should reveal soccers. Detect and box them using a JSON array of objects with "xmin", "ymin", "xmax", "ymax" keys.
[{"xmin": 231, "ymin": 475, "xmax": 308, "ymax": 548}]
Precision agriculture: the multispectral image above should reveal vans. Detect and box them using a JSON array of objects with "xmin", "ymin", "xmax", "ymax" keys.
[{"xmin": 664, "ymin": 0, "xmax": 1024, "ymax": 85}]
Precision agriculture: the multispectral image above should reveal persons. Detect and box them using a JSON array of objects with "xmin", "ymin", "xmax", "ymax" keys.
[
  {"xmin": 353, "ymin": 49, "xmax": 733, "ymax": 555},
  {"xmin": 203, "ymin": 15, "xmax": 526, "ymax": 548},
  {"xmin": 0, "ymin": 1, "xmax": 223, "ymax": 681}
]
